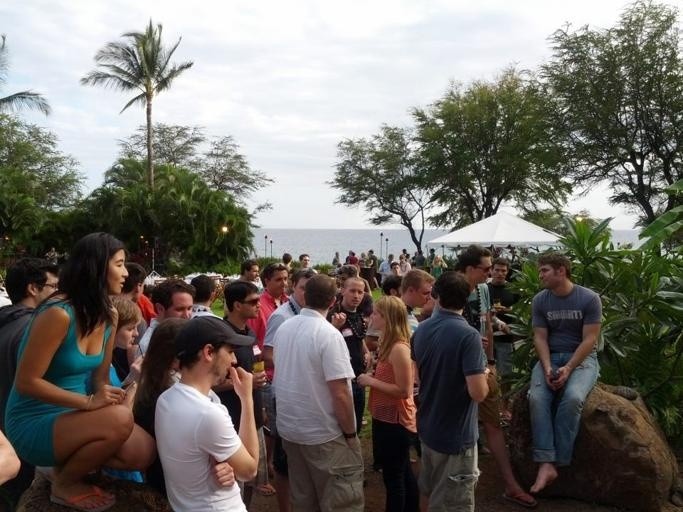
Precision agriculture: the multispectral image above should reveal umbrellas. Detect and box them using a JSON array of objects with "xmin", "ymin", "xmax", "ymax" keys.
[{"xmin": 428, "ymin": 208, "xmax": 566, "ymax": 246}]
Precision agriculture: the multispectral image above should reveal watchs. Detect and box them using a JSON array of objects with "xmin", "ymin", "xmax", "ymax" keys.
[{"xmin": 344, "ymin": 432, "xmax": 358, "ymax": 439}]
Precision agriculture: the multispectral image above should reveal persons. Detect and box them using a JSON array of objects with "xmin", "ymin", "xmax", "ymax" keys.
[
  {"xmin": 405, "ymin": 254, "xmax": 411, "ymax": 263},
  {"xmin": 0, "ymin": 257, "xmax": 59, "ymax": 431},
  {"xmin": 383, "ymin": 276, "xmax": 403, "ymax": 297},
  {"xmin": 221, "ymin": 281, "xmax": 276, "ymax": 494},
  {"xmin": 378, "ymin": 254, "xmax": 393, "ymax": 285},
  {"xmin": 528, "ymin": 254, "xmax": 601, "ymax": 492},
  {"xmin": 389, "ymin": 262, "xmax": 401, "ymax": 276},
  {"xmin": 131, "ymin": 316, "xmax": 188, "ymax": 490},
  {"xmin": 359, "ymin": 250, "xmax": 379, "ymax": 289},
  {"xmin": 426, "ymin": 248, "xmax": 436, "ymax": 271},
  {"xmin": 296, "ymin": 255, "xmax": 319, "ymax": 273},
  {"xmin": 412, "ymin": 250, "xmax": 426, "ymax": 268},
  {"xmin": 5, "ymin": 233, "xmax": 157, "ymax": 510},
  {"xmin": 358, "ymin": 295, "xmax": 418, "ymax": 512},
  {"xmin": 333, "ymin": 250, "xmax": 359, "ymax": 265},
  {"xmin": 247, "ymin": 263, "xmax": 291, "ymax": 381},
  {"xmin": 110, "ymin": 297, "xmax": 141, "ymax": 383},
  {"xmin": 272, "ymin": 275, "xmax": 365, "ymax": 511},
  {"xmin": 432, "ymin": 255, "xmax": 446, "ymax": 277},
  {"xmin": 0, "ymin": 431, "xmax": 21, "ymax": 486},
  {"xmin": 432, "ymin": 243, "xmax": 538, "ymax": 506},
  {"xmin": 156, "ymin": 317, "xmax": 260, "ymax": 511},
  {"xmin": 408, "ymin": 272, "xmax": 490, "ymax": 512},
  {"xmin": 493, "ymin": 247, "xmax": 504, "ymax": 258},
  {"xmin": 334, "ymin": 265, "xmax": 373, "ymax": 327},
  {"xmin": 486, "ymin": 258, "xmax": 521, "ymax": 421},
  {"xmin": 139, "ymin": 278, "xmax": 195, "ymax": 355},
  {"xmin": 262, "ymin": 270, "xmax": 316, "ymax": 369},
  {"xmin": 402, "ymin": 249, "xmax": 407, "ymax": 255},
  {"xmin": 133, "ymin": 294, "xmax": 155, "ymax": 344},
  {"xmin": 365, "ymin": 270, "xmax": 435, "ymax": 480},
  {"xmin": 223, "ymin": 259, "xmax": 264, "ymax": 318},
  {"xmin": 190, "ymin": 272, "xmax": 219, "ymax": 316},
  {"xmin": 399, "ymin": 255, "xmax": 411, "ymax": 276},
  {"xmin": 281, "ymin": 254, "xmax": 293, "ymax": 271},
  {"xmin": 121, "ymin": 262, "xmax": 147, "ymax": 302},
  {"xmin": 326, "ymin": 277, "xmax": 370, "ymax": 434}
]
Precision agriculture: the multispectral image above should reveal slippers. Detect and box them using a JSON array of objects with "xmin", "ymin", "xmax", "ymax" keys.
[
  {"xmin": 51, "ymin": 485, "xmax": 116, "ymax": 511},
  {"xmin": 503, "ymin": 488, "xmax": 537, "ymax": 508}
]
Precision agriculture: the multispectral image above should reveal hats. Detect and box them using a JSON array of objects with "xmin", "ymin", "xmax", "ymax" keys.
[{"xmin": 178, "ymin": 316, "xmax": 255, "ymax": 357}]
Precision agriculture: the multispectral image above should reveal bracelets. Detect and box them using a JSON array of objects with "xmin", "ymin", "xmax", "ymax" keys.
[{"xmin": 85, "ymin": 394, "xmax": 95, "ymax": 411}]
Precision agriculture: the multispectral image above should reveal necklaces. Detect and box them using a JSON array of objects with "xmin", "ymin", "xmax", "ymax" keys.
[
  {"xmin": 345, "ymin": 309, "xmax": 366, "ymax": 339},
  {"xmin": 466, "ymin": 286, "xmax": 481, "ymax": 327},
  {"xmin": 288, "ymin": 297, "xmax": 298, "ymax": 315}
]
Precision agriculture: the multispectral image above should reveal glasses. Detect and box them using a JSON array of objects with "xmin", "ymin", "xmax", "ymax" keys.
[
  {"xmin": 271, "ymin": 263, "xmax": 285, "ymax": 267},
  {"xmin": 473, "ymin": 265, "xmax": 493, "ymax": 272},
  {"xmin": 35, "ymin": 282, "xmax": 60, "ymax": 288},
  {"xmin": 238, "ymin": 297, "xmax": 260, "ymax": 306}
]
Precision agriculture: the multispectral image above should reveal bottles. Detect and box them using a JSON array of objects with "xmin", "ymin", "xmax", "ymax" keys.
[{"xmin": 252, "ymin": 345, "xmax": 264, "ymax": 385}]
